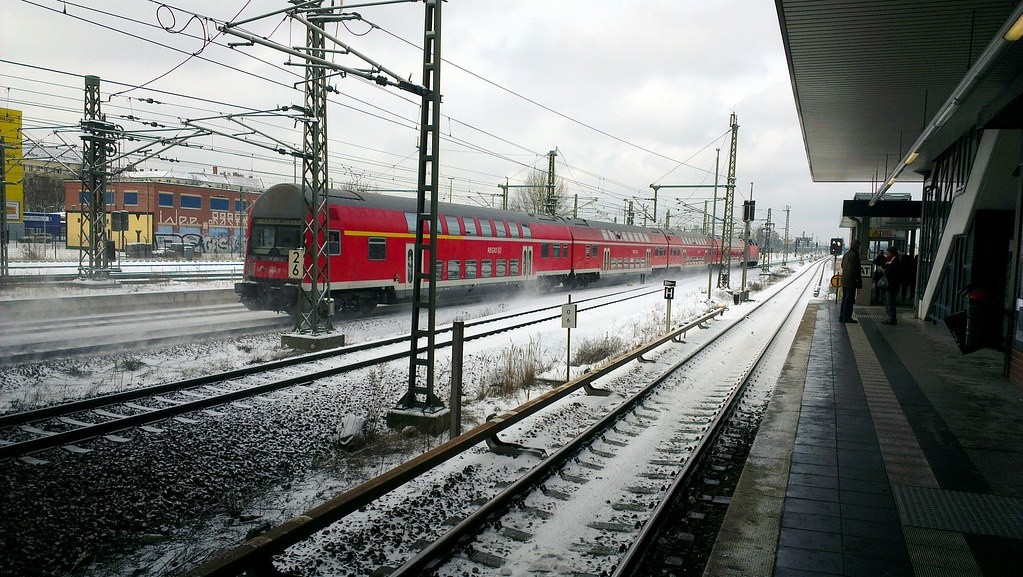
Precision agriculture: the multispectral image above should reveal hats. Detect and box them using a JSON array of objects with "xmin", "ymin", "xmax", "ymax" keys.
[{"xmin": 887, "ymin": 246, "xmax": 898, "ymax": 254}]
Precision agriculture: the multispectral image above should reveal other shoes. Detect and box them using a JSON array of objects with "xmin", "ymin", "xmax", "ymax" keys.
[
  {"xmin": 839, "ymin": 317, "xmax": 858, "ymax": 323},
  {"xmin": 882, "ymin": 320, "xmax": 897, "ymax": 325}
]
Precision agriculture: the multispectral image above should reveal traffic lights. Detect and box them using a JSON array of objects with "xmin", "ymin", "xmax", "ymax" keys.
[{"xmin": 829, "ymin": 238, "xmax": 843, "ymax": 256}]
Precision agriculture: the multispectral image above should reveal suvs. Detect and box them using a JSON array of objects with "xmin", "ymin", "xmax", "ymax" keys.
[{"xmin": 18, "ymin": 232, "xmax": 52, "ymax": 244}]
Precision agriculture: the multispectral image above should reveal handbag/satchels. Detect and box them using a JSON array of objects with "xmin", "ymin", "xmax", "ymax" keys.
[
  {"xmin": 855, "ymin": 276, "xmax": 862, "ymax": 289},
  {"xmin": 877, "ymin": 269, "xmax": 889, "ymax": 289}
]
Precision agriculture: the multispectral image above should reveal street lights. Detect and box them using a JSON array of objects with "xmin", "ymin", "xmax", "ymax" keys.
[{"xmin": 37, "ymin": 205, "xmax": 54, "ymax": 257}]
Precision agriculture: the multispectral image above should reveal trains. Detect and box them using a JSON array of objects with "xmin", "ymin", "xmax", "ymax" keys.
[{"xmin": 231, "ymin": 177, "xmax": 762, "ymax": 321}]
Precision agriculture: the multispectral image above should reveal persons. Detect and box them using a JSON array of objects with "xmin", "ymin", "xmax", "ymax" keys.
[
  {"xmin": 871, "ymin": 250, "xmax": 918, "ymax": 309},
  {"xmin": 882, "ymin": 246, "xmax": 901, "ymax": 325},
  {"xmin": 839, "ymin": 239, "xmax": 862, "ymax": 323}
]
